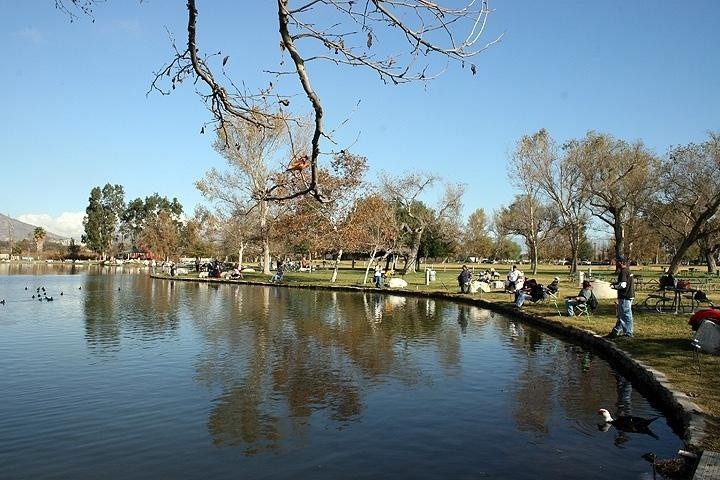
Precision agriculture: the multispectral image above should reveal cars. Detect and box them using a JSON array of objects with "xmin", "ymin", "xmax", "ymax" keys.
[{"xmin": 480, "ymin": 257, "xmax": 651, "ymax": 265}]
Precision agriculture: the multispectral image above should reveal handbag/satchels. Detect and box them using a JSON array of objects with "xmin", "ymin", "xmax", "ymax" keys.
[
  {"xmin": 655, "ymin": 274, "xmax": 675, "ymax": 292},
  {"xmin": 695, "ymin": 291, "xmax": 706, "ymax": 300}
]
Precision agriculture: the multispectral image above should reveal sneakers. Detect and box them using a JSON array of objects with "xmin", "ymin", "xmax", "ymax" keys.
[{"xmin": 602, "ymin": 332, "xmax": 616, "ymax": 339}]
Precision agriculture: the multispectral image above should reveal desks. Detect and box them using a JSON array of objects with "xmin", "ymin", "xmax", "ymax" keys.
[
  {"xmin": 466, "ymin": 281, "xmax": 490, "ymax": 298},
  {"xmin": 659, "ymin": 286, "xmax": 697, "ymax": 315}
]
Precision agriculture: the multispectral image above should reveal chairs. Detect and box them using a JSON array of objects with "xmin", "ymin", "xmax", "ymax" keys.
[
  {"xmin": 547, "ymin": 290, "xmax": 560, "ymax": 302},
  {"xmin": 575, "ymin": 297, "xmax": 592, "ymax": 317},
  {"xmin": 505, "ymin": 271, "xmax": 524, "ymax": 293}
]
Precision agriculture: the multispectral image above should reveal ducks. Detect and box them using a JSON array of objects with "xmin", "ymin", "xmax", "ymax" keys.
[{"xmin": 596, "ymin": 407, "xmax": 661, "ymax": 427}]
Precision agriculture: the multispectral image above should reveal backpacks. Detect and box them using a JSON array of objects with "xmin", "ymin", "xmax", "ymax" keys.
[{"xmin": 590, "ymin": 290, "xmax": 598, "ymax": 311}]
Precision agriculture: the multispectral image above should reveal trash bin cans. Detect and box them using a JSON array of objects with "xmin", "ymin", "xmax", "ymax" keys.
[{"xmin": 426, "ymin": 270, "xmax": 436, "ymax": 286}]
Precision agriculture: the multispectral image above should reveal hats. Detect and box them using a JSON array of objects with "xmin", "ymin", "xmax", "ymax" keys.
[
  {"xmin": 581, "ymin": 281, "xmax": 591, "ymax": 286},
  {"xmin": 553, "ymin": 277, "xmax": 560, "ymax": 284},
  {"xmin": 615, "ymin": 255, "xmax": 627, "ymax": 262}
]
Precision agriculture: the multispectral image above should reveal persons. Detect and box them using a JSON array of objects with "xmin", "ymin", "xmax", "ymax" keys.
[
  {"xmin": 195, "ymin": 256, "xmax": 200, "ymax": 272},
  {"xmin": 170, "ymin": 260, "xmax": 176, "ymax": 277},
  {"xmin": 604, "ymin": 255, "xmax": 635, "ymax": 338},
  {"xmin": 373, "ymin": 260, "xmax": 381, "ymax": 283},
  {"xmin": 457, "ymin": 265, "xmax": 470, "ymax": 294},
  {"xmin": 374, "ymin": 264, "xmax": 385, "ymax": 288},
  {"xmin": 560, "ymin": 280, "xmax": 593, "ymax": 316},
  {"xmin": 506, "ymin": 265, "xmax": 521, "ymax": 293},
  {"xmin": 269, "ymin": 264, "xmax": 283, "ymax": 284},
  {"xmin": 512, "ymin": 279, "xmax": 542, "ymax": 309}
]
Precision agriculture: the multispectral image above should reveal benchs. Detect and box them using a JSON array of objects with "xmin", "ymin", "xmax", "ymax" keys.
[
  {"xmin": 645, "ymin": 294, "xmax": 672, "ymax": 314},
  {"xmin": 682, "ymin": 294, "xmax": 714, "ymax": 312}
]
